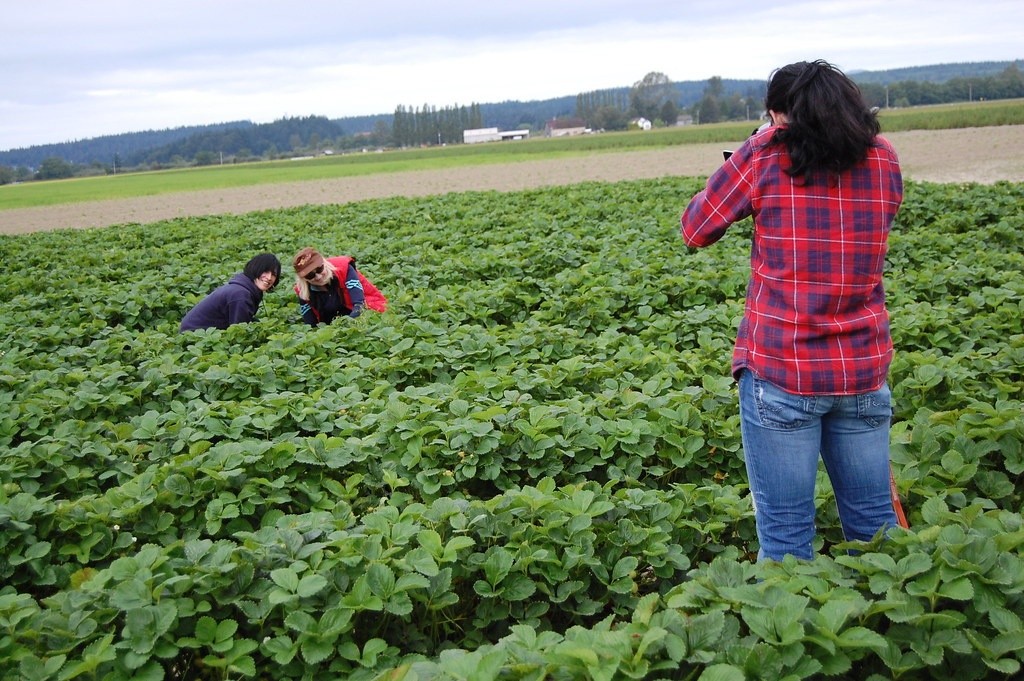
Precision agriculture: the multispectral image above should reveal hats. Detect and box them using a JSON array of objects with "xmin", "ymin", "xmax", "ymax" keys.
[{"xmin": 292, "ymin": 247, "xmax": 321, "ymax": 273}]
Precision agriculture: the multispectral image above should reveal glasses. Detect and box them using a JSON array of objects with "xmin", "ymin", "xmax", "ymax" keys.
[{"xmin": 301, "ymin": 264, "xmax": 324, "ymax": 280}]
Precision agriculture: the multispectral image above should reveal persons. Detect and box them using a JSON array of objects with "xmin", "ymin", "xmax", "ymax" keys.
[
  {"xmin": 293, "ymin": 247, "xmax": 389, "ymax": 331},
  {"xmin": 682, "ymin": 57, "xmax": 905, "ymax": 565},
  {"xmin": 179, "ymin": 253, "xmax": 282, "ymax": 333}
]
